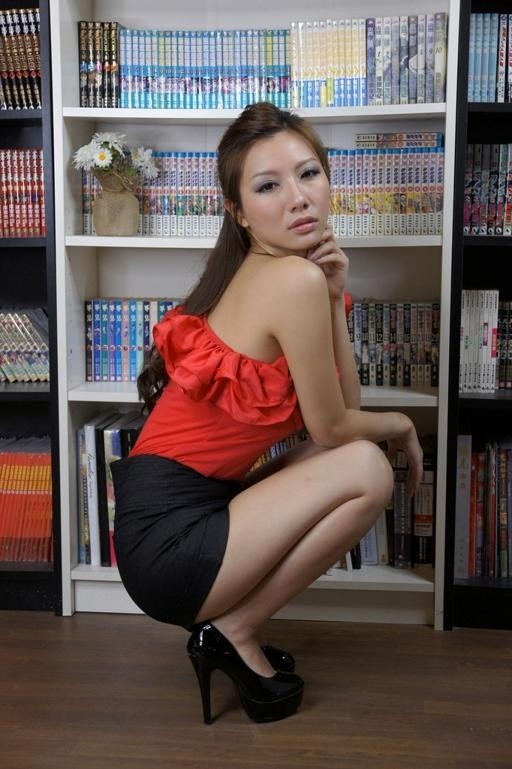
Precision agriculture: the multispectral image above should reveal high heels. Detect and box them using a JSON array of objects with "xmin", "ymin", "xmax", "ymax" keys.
[
  {"xmin": 187, "ymin": 621, "xmax": 308, "ymax": 728},
  {"xmin": 258, "ymin": 639, "xmax": 297, "ymax": 673}
]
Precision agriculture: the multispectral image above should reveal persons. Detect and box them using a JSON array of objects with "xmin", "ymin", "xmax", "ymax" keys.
[{"xmin": 109, "ymin": 102, "xmax": 428, "ymax": 725}]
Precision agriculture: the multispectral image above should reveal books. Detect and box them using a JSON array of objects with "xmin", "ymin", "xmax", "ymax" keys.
[
  {"xmin": 0, "ymin": 7, "xmax": 56, "ymax": 570},
  {"xmin": 82, "ymin": 132, "xmax": 444, "ymax": 237},
  {"xmin": 77, "ymin": 13, "xmax": 448, "ymax": 109},
  {"xmin": 76, "ymin": 408, "xmax": 436, "ymax": 571},
  {"xmin": 454, "ymin": 14, "xmax": 510, "ymax": 583},
  {"xmin": 85, "ymin": 297, "xmax": 440, "ymax": 387}
]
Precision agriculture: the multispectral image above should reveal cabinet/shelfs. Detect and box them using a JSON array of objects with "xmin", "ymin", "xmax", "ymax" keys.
[
  {"xmin": 50, "ymin": 0, "xmax": 455, "ymax": 626},
  {"xmin": 451, "ymin": 0, "xmax": 512, "ymax": 607},
  {"xmin": 1, "ymin": 0, "xmax": 51, "ymax": 577}
]
